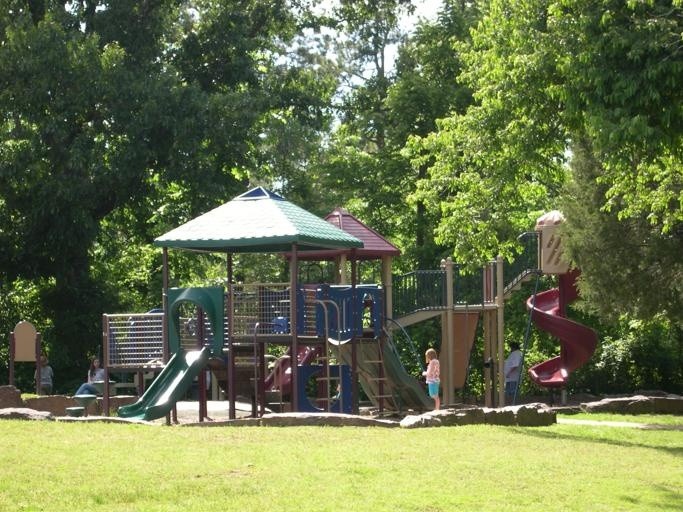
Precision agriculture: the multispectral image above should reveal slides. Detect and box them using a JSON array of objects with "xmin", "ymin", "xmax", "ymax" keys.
[
  {"xmin": 327, "ymin": 329, "xmax": 435, "ymax": 411},
  {"xmin": 117, "ymin": 346, "xmax": 214, "ymax": 420},
  {"xmin": 526, "ymin": 268, "xmax": 597, "ymax": 386},
  {"xmin": 260, "ymin": 344, "xmax": 317, "ymax": 396}
]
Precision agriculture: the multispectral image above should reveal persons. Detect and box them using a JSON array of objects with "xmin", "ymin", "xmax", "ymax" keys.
[
  {"xmin": 74, "ymin": 357, "xmax": 110, "ymax": 395},
  {"xmin": 332, "ymin": 384, "xmax": 340, "ymax": 399},
  {"xmin": 421, "ymin": 348, "xmax": 441, "ymax": 411},
  {"xmin": 363, "ymin": 298, "xmax": 372, "ymax": 309},
  {"xmin": 503, "ymin": 341, "xmax": 523, "ymax": 405},
  {"xmin": 34, "ymin": 356, "xmax": 54, "ymax": 395}
]
